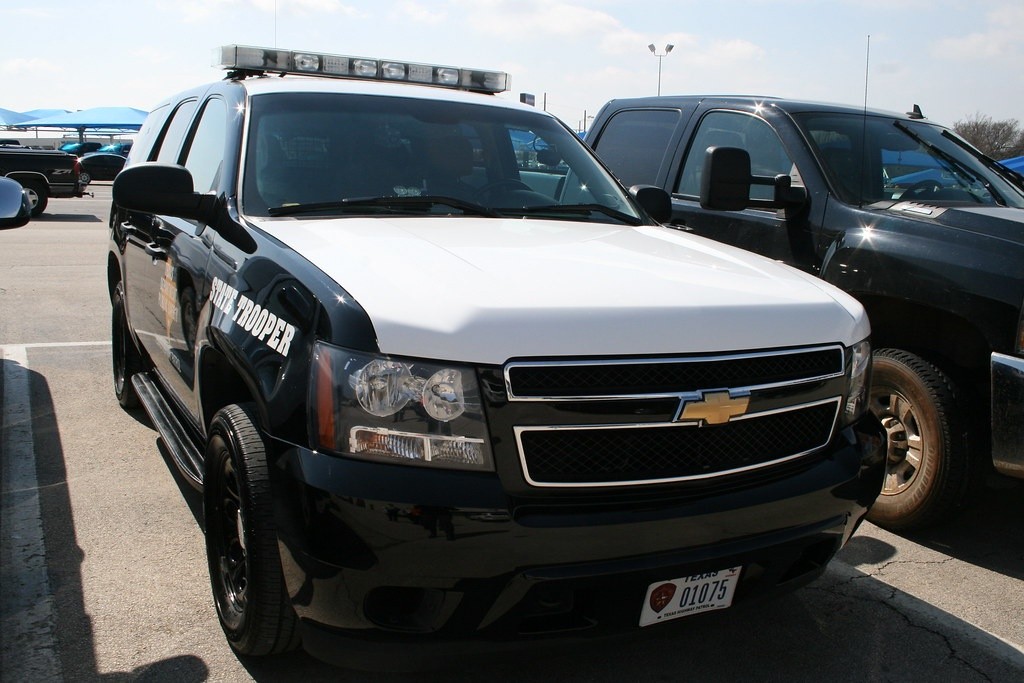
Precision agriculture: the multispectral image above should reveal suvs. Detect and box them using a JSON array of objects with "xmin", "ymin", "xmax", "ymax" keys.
[{"xmin": 105, "ymin": 44, "xmax": 890, "ymax": 671}]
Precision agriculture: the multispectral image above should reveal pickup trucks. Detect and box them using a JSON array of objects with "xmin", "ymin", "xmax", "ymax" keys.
[{"xmin": 522, "ymin": 95, "xmax": 1024, "ymax": 541}]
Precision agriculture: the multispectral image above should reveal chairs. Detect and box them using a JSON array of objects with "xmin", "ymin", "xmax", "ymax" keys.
[
  {"xmin": 697, "ymin": 130, "xmax": 743, "ymax": 159},
  {"xmin": 821, "ymin": 148, "xmax": 873, "ymax": 193},
  {"xmin": 411, "ymin": 127, "xmax": 485, "ymax": 206},
  {"xmin": 258, "ymin": 134, "xmax": 313, "ymax": 200}
]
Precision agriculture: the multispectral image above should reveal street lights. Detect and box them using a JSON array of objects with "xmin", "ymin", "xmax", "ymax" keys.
[{"xmin": 648, "ymin": 44, "xmax": 674, "ymax": 97}]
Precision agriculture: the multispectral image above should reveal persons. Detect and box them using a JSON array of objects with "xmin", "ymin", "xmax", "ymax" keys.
[
  {"xmin": 347, "ymin": 495, "xmax": 361, "ymax": 506},
  {"xmin": 392, "ymin": 407, "xmax": 404, "ymax": 424},
  {"xmin": 386, "ymin": 503, "xmax": 400, "ymax": 522},
  {"xmin": 428, "ymin": 416, "xmax": 451, "ymax": 437},
  {"xmin": 405, "ymin": 505, "xmax": 421, "ymax": 524},
  {"xmin": 368, "ymin": 504, "xmax": 374, "ymax": 512},
  {"xmin": 416, "ymin": 497, "xmax": 455, "ymax": 541}
]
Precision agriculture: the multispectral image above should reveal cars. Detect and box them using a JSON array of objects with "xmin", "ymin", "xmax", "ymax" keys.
[
  {"xmin": 0, "ymin": 140, "xmax": 132, "ymax": 230},
  {"xmin": 468, "ymin": 131, "xmax": 586, "ymax": 174},
  {"xmin": 879, "ymin": 142, "xmax": 1024, "ymax": 204}
]
